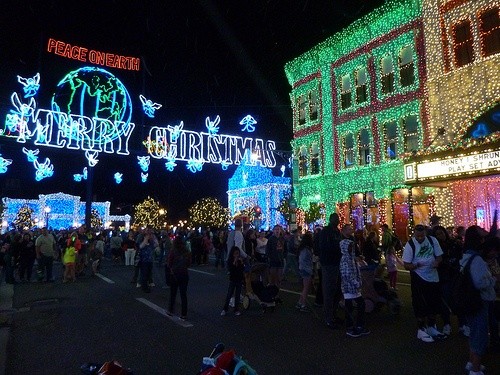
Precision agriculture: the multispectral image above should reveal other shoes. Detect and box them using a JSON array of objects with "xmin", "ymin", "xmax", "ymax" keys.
[
  {"xmin": 200, "ymin": 342, "xmax": 259, "ymax": 375},
  {"xmin": 470, "ymin": 369, "xmax": 484, "ymax": 375},
  {"xmin": 465, "ymin": 361, "xmax": 486, "ymax": 371},
  {"xmin": 136, "ymin": 282, "xmax": 142, "ymax": 288},
  {"xmin": 295, "ymin": 301, "xmax": 311, "ymax": 313},
  {"xmin": 147, "ymin": 282, "xmax": 155, "ymax": 287},
  {"xmin": 345, "ymin": 325, "xmax": 370, "ymax": 337},
  {"xmin": 314, "ymin": 299, "xmax": 325, "ymax": 306},
  {"xmin": 37, "ymin": 272, "xmax": 100, "ymax": 284},
  {"xmin": 164, "ymin": 309, "xmax": 188, "ymax": 321},
  {"xmin": 324, "ymin": 319, "xmax": 337, "ymax": 329},
  {"xmin": 191, "ymin": 262, "xmax": 224, "ymax": 271},
  {"xmin": 389, "ymin": 286, "xmax": 400, "ymax": 291}
]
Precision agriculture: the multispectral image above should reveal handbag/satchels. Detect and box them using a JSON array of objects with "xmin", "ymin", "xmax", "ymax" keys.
[
  {"xmin": 165, "ymin": 263, "xmax": 173, "ymax": 276},
  {"xmin": 121, "ymin": 243, "xmax": 128, "ymax": 250}
]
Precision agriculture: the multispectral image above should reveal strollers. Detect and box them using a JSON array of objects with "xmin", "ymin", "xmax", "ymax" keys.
[{"xmin": 240, "ymin": 255, "xmax": 280, "ymax": 310}]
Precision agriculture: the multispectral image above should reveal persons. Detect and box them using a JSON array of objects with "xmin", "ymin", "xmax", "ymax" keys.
[
  {"xmin": 0, "ymin": 230, "xmax": 56, "ymax": 284},
  {"xmin": 35, "ymin": 227, "xmax": 56, "ymax": 281},
  {"xmin": 166, "ymin": 236, "xmax": 190, "ymax": 321},
  {"xmin": 403, "ymin": 224, "xmax": 500, "ymax": 375},
  {"xmin": 386, "ymin": 246, "xmax": 399, "ymax": 290},
  {"xmin": 225, "ymin": 218, "xmax": 288, "ymax": 310},
  {"xmin": 123, "ymin": 223, "xmax": 174, "ymax": 294},
  {"xmin": 220, "ymin": 245, "xmax": 244, "ymax": 315},
  {"xmin": 339, "ymin": 239, "xmax": 370, "ymax": 337},
  {"xmin": 381, "ymin": 224, "xmax": 395, "ymax": 279},
  {"xmin": 286, "ymin": 213, "xmax": 382, "ymax": 329},
  {"xmin": 174, "ymin": 226, "xmax": 230, "ymax": 271},
  {"xmin": 58, "ymin": 227, "xmax": 125, "ymax": 284}
]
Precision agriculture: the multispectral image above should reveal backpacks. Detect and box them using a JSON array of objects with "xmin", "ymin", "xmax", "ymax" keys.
[
  {"xmin": 385, "ymin": 231, "xmax": 403, "ymax": 251},
  {"xmin": 443, "ymin": 253, "xmax": 489, "ymax": 316}
]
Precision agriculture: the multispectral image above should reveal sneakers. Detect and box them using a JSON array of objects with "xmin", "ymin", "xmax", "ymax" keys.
[
  {"xmin": 426, "ymin": 325, "xmax": 447, "ymax": 339},
  {"xmin": 416, "ymin": 327, "xmax": 434, "ymax": 342},
  {"xmin": 459, "ymin": 324, "xmax": 470, "ymax": 336},
  {"xmin": 434, "ymin": 323, "xmax": 452, "ymax": 335}
]
